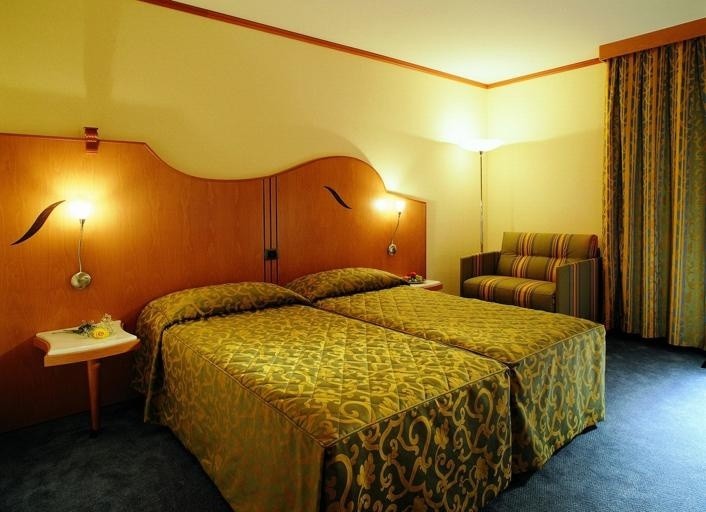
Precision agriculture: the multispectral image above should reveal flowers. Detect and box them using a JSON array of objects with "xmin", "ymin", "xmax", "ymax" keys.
[{"xmin": 74, "ymin": 313, "xmax": 113, "ymax": 339}]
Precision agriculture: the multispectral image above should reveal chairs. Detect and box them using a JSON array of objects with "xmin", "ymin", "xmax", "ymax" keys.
[{"xmin": 459, "ymin": 232, "xmax": 605, "ymax": 325}]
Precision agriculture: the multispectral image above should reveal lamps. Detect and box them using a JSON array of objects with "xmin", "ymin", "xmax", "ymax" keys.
[
  {"xmin": 386, "ymin": 211, "xmax": 403, "ymax": 258},
  {"xmin": 70, "ymin": 217, "xmax": 93, "ymax": 289}
]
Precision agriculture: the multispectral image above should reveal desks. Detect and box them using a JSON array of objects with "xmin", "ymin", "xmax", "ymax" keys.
[
  {"xmin": 409, "ymin": 278, "xmax": 443, "ymax": 291},
  {"xmin": 32, "ymin": 319, "xmax": 142, "ymax": 432}
]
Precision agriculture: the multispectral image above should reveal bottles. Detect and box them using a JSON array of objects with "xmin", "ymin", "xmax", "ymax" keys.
[{"xmin": 410, "ymin": 272, "xmax": 416, "ymax": 282}]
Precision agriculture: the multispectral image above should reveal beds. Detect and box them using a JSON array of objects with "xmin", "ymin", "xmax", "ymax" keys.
[
  {"xmin": 126, "ymin": 304, "xmax": 513, "ymax": 512},
  {"xmin": 310, "ymin": 284, "xmax": 607, "ymax": 475}
]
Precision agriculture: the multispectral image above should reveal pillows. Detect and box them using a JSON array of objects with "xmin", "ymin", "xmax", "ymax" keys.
[
  {"xmin": 283, "ymin": 266, "xmax": 410, "ymax": 304},
  {"xmin": 144, "ymin": 279, "xmax": 310, "ymax": 327}
]
